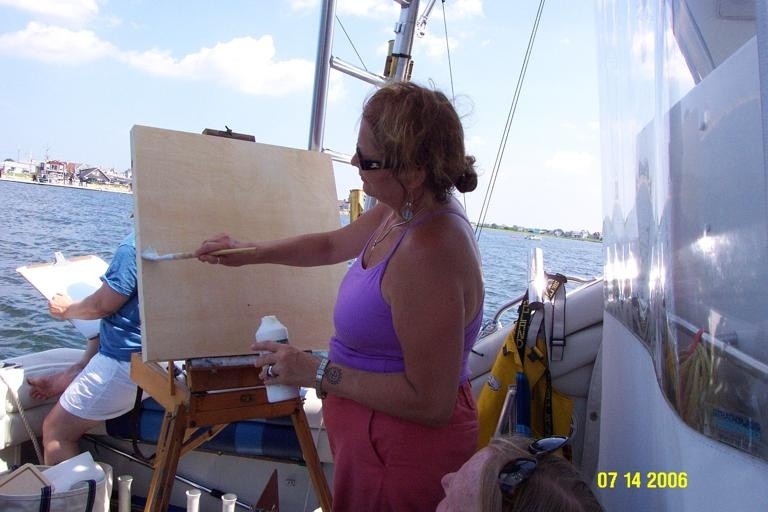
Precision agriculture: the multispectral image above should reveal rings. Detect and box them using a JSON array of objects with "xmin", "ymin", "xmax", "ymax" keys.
[
  {"xmin": 217, "ymin": 258, "xmax": 220, "ymax": 265},
  {"xmin": 266, "ymin": 365, "xmax": 275, "ymax": 378}
]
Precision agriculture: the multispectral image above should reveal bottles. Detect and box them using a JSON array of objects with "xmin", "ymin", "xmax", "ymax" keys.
[
  {"xmin": 221, "ymin": 493, "xmax": 238, "ymax": 512},
  {"xmin": 117, "ymin": 474, "xmax": 134, "ymax": 512},
  {"xmin": 185, "ymin": 489, "xmax": 202, "ymax": 512},
  {"xmin": 254, "ymin": 315, "xmax": 300, "ymax": 403}
]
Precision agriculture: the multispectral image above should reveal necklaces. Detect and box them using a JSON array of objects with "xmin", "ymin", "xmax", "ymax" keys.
[{"xmin": 371, "ymin": 209, "xmax": 426, "ymax": 250}]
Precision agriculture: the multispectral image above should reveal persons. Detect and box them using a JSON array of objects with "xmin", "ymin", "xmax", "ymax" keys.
[
  {"xmin": 25, "ymin": 229, "xmax": 186, "ymax": 466},
  {"xmin": 435, "ymin": 435, "xmax": 603, "ymax": 512},
  {"xmin": 195, "ymin": 81, "xmax": 485, "ymax": 512}
]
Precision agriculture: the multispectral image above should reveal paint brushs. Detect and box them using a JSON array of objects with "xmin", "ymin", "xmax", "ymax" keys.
[{"xmin": 141, "ymin": 245, "xmax": 257, "ymax": 262}]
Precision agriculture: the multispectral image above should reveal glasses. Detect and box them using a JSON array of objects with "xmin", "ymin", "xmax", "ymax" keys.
[
  {"xmin": 354, "ymin": 147, "xmax": 386, "ymax": 172},
  {"xmin": 495, "ymin": 432, "xmax": 574, "ymax": 512}
]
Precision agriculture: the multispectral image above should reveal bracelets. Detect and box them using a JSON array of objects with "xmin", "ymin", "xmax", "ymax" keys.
[{"xmin": 316, "ymin": 359, "xmax": 330, "ymax": 399}]
